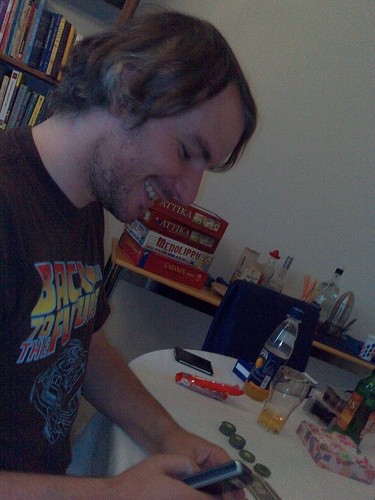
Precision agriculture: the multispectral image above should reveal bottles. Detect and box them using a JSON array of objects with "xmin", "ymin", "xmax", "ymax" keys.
[
  {"xmin": 255, "ymin": 249, "xmax": 281, "ymax": 286},
  {"xmin": 268, "ymin": 256, "xmax": 294, "ymax": 292},
  {"xmin": 310, "ymin": 264, "xmax": 345, "ymax": 323},
  {"xmin": 242, "ymin": 307, "xmax": 305, "ymax": 403},
  {"xmin": 326, "ymin": 368, "xmax": 375, "ymax": 449}
]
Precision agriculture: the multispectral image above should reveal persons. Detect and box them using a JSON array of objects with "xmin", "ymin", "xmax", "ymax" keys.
[{"xmin": 0, "ymin": 11, "xmax": 258, "ymax": 500}]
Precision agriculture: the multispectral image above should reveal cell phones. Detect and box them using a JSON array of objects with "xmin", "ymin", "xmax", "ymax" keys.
[
  {"xmin": 173, "ymin": 346, "xmax": 213, "ymax": 376},
  {"xmin": 181, "ymin": 460, "xmax": 252, "ymax": 495}
]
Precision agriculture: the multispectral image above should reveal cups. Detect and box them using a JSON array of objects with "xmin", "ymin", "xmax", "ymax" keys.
[
  {"xmin": 359, "ymin": 329, "xmax": 375, "ymax": 361},
  {"xmin": 238, "ymin": 262, "xmax": 264, "ymax": 285},
  {"xmin": 257, "ymin": 365, "xmax": 310, "ymax": 436},
  {"xmin": 229, "ymin": 246, "xmax": 261, "ymax": 286}
]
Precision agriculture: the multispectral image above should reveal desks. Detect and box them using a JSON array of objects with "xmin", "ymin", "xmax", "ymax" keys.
[
  {"xmin": 66, "ymin": 348, "xmax": 375, "ymax": 500},
  {"xmin": 103, "ymin": 238, "xmax": 375, "ymax": 370}
]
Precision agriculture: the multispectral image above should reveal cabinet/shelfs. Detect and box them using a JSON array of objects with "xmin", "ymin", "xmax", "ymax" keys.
[{"xmin": 0, "ymin": 0, "xmax": 140, "ymax": 130}]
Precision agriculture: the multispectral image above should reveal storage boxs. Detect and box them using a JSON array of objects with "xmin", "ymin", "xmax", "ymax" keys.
[
  {"xmin": 297, "ymin": 420, "xmax": 375, "ymax": 484},
  {"xmin": 229, "ymin": 247, "xmax": 261, "ymax": 284},
  {"xmin": 118, "ymin": 195, "xmax": 229, "ymax": 288},
  {"xmin": 233, "ymin": 357, "xmax": 253, "ymax": 381}
]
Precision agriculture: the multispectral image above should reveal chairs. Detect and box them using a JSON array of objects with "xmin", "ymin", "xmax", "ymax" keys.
[{"xmin": 202, "ymin": 280, "xmax": 321, "ymax": 371}]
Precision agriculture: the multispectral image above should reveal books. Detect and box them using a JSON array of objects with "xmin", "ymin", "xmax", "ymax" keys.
[{"xmin": 0, "ymin": 0, "xmax": 83, "ymax": 129}]
